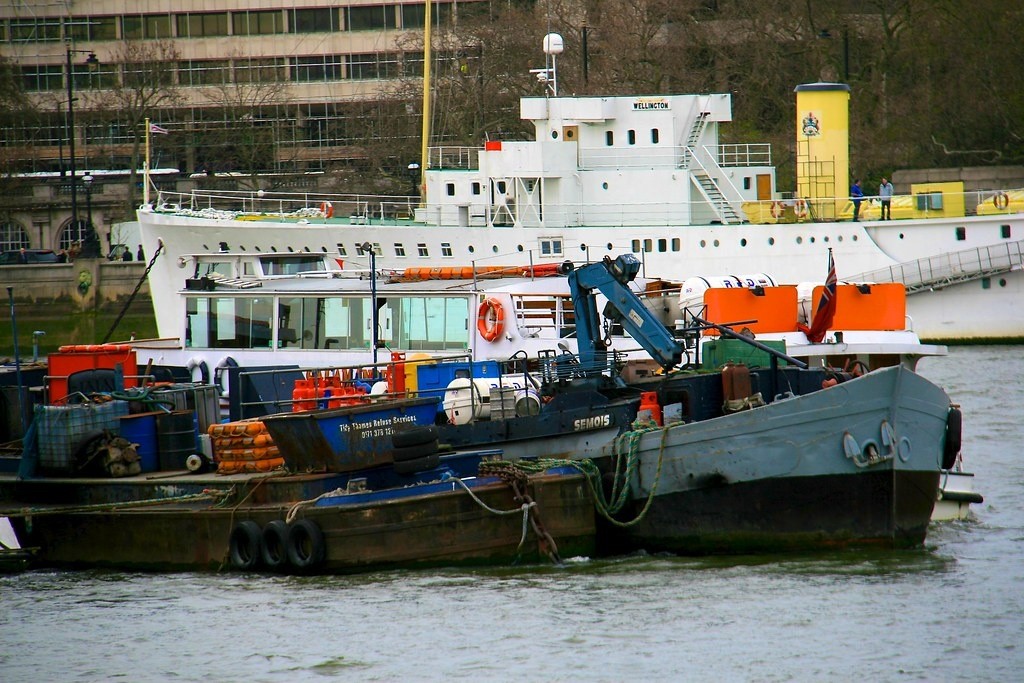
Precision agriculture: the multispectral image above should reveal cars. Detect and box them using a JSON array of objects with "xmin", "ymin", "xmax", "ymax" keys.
[{"xmin": 0, "ymin": 248, "xmax": 59, "ymax": 265}]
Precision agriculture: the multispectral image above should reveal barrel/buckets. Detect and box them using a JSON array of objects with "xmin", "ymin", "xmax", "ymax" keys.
[
  {"xmin": 198, "ymin": 433, "xmax": 213, "ymax": 459},
  {"xmin": 415, "ymin": 467, "xmax": 454, "ymax": 486},
  {"xmin": 156, "ymin": 409, "xmax": 196, "ymax": 471},
  {"xmin": 515, "ymin": 390, "xmax": 541, "ymax": 418},
  {"xmin": 119, "ymin": 415, "xmax": 156, "ymax": 473},
  {"xmin": 488, "ymin": 387, "xmax": 515, "ymax": 420}
]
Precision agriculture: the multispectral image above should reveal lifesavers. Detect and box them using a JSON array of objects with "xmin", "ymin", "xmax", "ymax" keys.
[
  {"xmin": 58, "ymin": 343, "xmax": 132, "ymax": 352},
  {"xmin": 391, "ymin": 426, "xmax": 440, "ymax": 474},
  {"xmin": 214, "ymin": 355, "xmax": 239, "ymax": 398},
  {"xmin": 259, "ymin": 519, "xmax": 290, "ymax": 571},
  {"xmin": 794, "ymin": 200, "xmax": 808, "ymax": 216},
  {"xmin": 321, "ymin": 200, "xmax": 333, "ymax": 218},
  {"xmin": 522, "ymin": 263, "xmax": 559, "ymax": 277},
  {"xmin": 287, "ymin": 519, "xmax": 325, "ymax": 570},
  {"xmin": 994, "ymin": 192, "xmax": 1009, "ymax": 209},
  {"xmin": 228, "ymin": 520, "xmax": 263, "ymax": 570},
  {"xmin": 770, "ymin": 201, "xmax": 784, "ymax": 218},
  {"xmin": 477, "ymin": 296, "xmax": 504, "ymax": 341},
  {"xmin": 943, "ymin": 409, "xmax": 962, "ymax": 470},
  {"xmin": 186, "ymin": 357, "xmax": 209, "ymax": 384}
]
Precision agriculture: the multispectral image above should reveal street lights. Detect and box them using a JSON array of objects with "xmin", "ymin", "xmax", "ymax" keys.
[{"xmin": 66, "ymin": 47, "xmax": 100, "ymax": 244}]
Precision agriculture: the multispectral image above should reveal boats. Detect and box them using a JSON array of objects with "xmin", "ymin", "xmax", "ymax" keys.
[
  {"xmin": 135, "ymin": 0, "xmax": 1024, "ymax": 351},
  {"xmin": 0, "ymin": 242, "xmax": 987, "ymax": 580}
]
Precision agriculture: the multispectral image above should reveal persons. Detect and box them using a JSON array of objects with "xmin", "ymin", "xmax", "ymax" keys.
[
  {"xmin": 850, "ymin": 178, "xmax": 863, "ymax": 222},
  {"xmin": 136, "ymin": 244, "xmax": 144, "ymax": 260},
  {"xmin": 877, "ymin": 176, "xmax": 893, "ymax": 220},
  {"xmin": 122, "ymin": 246, "xmax": 132, "ymax": 260}
]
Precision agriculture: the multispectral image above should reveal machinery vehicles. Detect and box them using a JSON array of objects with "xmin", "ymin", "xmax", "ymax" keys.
[{"xmin": 539, "ymin": 249, "xmax": 689, "ymax": 403}]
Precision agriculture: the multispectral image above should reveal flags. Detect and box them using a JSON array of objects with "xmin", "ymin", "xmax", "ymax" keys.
[
  {"xmin": 796, "ymin": 253, "xmax": 837, "ymax": 342},
  {"xmin": 148, "ymin": 122, "xmax": 168, "ymax": 134}
]
